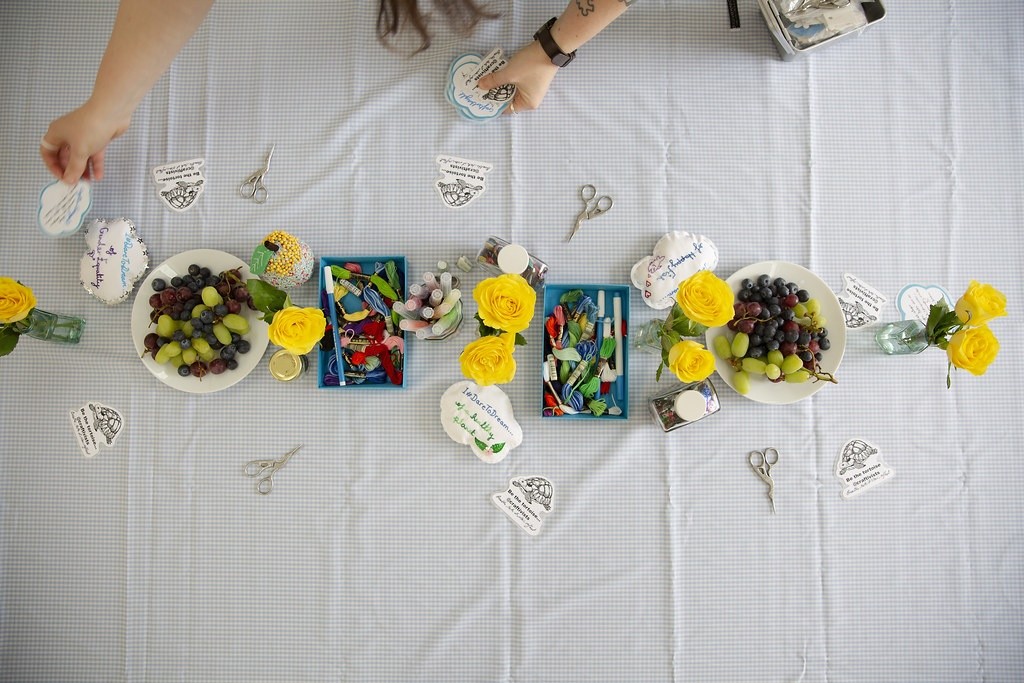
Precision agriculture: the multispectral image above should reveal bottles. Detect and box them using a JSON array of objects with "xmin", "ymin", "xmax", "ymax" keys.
[
  {"xmin": 271, "ymin": 350, "xmax": 309, "ymax": 381},
  {"xmin": 477, "ymin": 237, "xmax": 548, "ymax": 294},
  {"xmin": 651, "ymin": 378, "xmax": 719, "ymax": 431}
]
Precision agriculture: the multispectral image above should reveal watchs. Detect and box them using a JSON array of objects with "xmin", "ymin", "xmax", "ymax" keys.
[{"xmin": 534, "ymin": 17, "xmax": 577, "ymax": 66}]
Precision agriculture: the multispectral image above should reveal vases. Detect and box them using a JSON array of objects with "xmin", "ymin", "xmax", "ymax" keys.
[
  {"xmin": 634, "ymin": 318, "xmax": 669, "ymax": 355},
  {"xmin": 21, "ymin": 307, "xmax": 87, "ymax": 345},
  {"xmin": 875, "ymin": 317, "xmax": 932, "ymax": 357}
]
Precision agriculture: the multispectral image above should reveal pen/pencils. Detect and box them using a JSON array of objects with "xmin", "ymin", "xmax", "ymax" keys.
[
  {"xmin": 324, "ymin": 266, "xmax": 346, "ymax": 387},
  {"xmin": 727, "ymin": 0, "xmax": 741, "ymax": 29},
  {"xmin": 594, "ymin": 290, "xmax": 605, "ymax": 399},
  {"xmin": 613, "ymin": 292, "xmax": 625, "ymax": 400}
]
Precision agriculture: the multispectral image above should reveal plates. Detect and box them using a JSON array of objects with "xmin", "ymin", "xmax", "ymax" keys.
[
  {"xmin": 704, "ymin": 260, "xmax": 847, "ymax": 405},
  {"xmin": 131, "ymin": 249, "xmax": 270, "ymax": 394}
]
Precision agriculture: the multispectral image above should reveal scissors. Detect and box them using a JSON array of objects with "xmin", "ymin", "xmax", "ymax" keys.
[
  {"xmin": 748, "ymin": 447, "xmax": 778, "ymax": 514},
  {"xmin": 788, "ymin": 0, "xmax": 851, "ymax": 15},
  {"xmin": 239, "ymin": 144, "xmax": 275, "ymax": 203},
  {"xmin": 567, "ymin": 184, "xmax": 613, "ymax": 243},
  {"xmin": 244, "ymin": 444, "xmax": 303, "ymax": 495}
]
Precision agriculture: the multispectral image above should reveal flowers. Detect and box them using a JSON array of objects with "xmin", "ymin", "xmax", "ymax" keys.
[
  {"xmin": 635, "ymin": 269, "xmax": 735, "ymax": 384},
  {"xmin": 0, "ymin": 276, "xmax": 81, "ymax": 356},
  {"xmin": 246, "ymin": 278, "xmax": 327, "ymax": 356},
  {"xmin": 897, "ymin": 279, "xmax": 1008, "ymax": 388},
  {"xmin": 459, "ymin": 273, "xmax": 537, "ymax": 387}
]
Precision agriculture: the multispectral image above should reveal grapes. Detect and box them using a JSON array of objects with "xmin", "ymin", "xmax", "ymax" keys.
[
  {"xmin": 142, "ymin": 267, "xmax": 258, "ymax": 379},
  {"xmin": 713, "ymin": 295, "xmax": 830, "ymax": 395}
]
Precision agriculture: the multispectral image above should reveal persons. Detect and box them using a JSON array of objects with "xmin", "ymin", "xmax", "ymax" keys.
[{"xmin": 38, "ymin": 0, "xmax": 638, "ymax": 185}]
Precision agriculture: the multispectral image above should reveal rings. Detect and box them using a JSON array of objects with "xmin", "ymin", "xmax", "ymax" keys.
[
  {"xmin": 510, "ymin": 104, "xmax": 518, "ymax": 115},
  {"xmin": 42, "ymin": 140, "xmax": 59, "ymax": 151}
]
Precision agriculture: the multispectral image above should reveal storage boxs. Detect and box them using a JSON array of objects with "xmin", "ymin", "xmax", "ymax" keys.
[
  {"xmin": 757, "ymin": 0, "xmax": 887, "ymax": 63},
  {"xmin": 317, "ymin": 254, "xmax": 408, "ymax": 390},
  {"xmin": 540, "ymin": 284, "xmax": 631, "ymax": 422}
]
